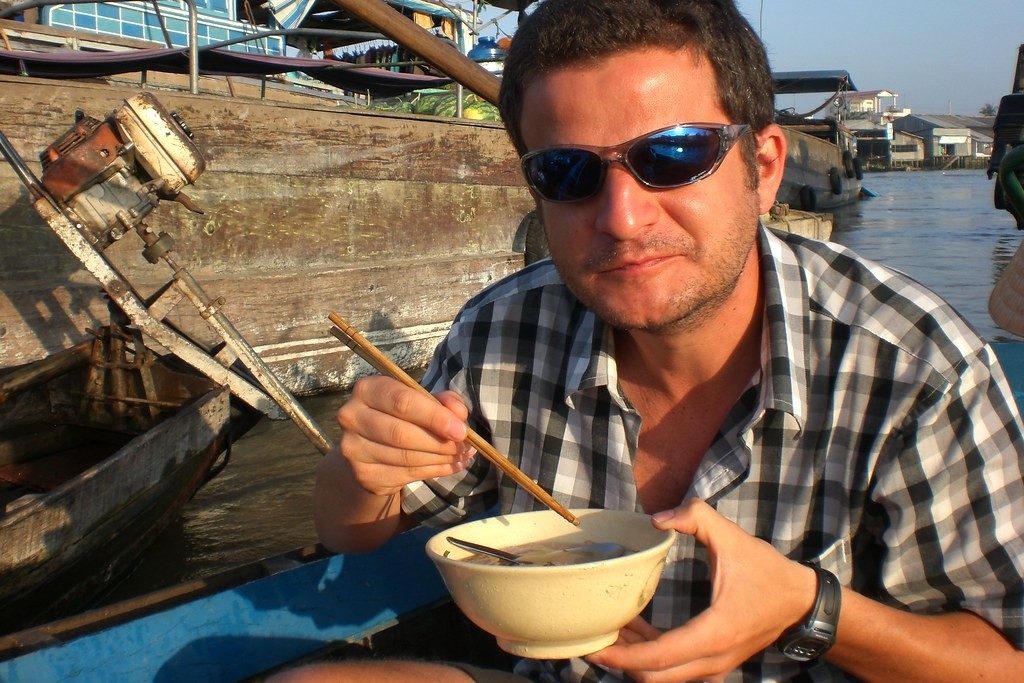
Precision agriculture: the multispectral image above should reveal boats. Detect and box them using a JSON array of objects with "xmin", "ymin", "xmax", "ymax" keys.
[
  {"xmin": 0, "ymin": 1, "xmax": 830, "ymax": 462},
  {"xmin": 761, "ymin": 69, "xmax": 864, "ymax": 213},
  {"xmin": 0, "ymin": 329, "xmax": 236, "ymax": 619}
]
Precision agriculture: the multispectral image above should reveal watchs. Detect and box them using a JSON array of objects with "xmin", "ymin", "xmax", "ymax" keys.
[{"xmin": 777, "ymin": 560, "xmax": 841, "ymax": 662}]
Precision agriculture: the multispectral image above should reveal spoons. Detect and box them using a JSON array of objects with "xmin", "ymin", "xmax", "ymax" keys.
[{"xmin": 445, "ymin": 536, "xmax": 624, "ymax": 567}]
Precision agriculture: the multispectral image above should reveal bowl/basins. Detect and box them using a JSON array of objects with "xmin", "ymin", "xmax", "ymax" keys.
[{"xmin": 425, "ymin": 509, "xmax": 676, "ymax": 660}]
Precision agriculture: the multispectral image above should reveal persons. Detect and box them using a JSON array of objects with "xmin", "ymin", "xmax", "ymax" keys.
[{"xmin": 264, "ymin": 0, "xmax": 1024, "ymax": 683}]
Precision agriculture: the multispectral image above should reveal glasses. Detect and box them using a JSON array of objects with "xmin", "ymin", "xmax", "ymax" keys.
[{"xmin": 520, "ymin": 122, "xmax": 753, "ymax": 205}]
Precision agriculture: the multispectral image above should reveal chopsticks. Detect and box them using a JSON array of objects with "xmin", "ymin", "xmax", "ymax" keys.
[{"xmin": 327, "ymin": 310, "xmax": 580, "ymax": 526}]
